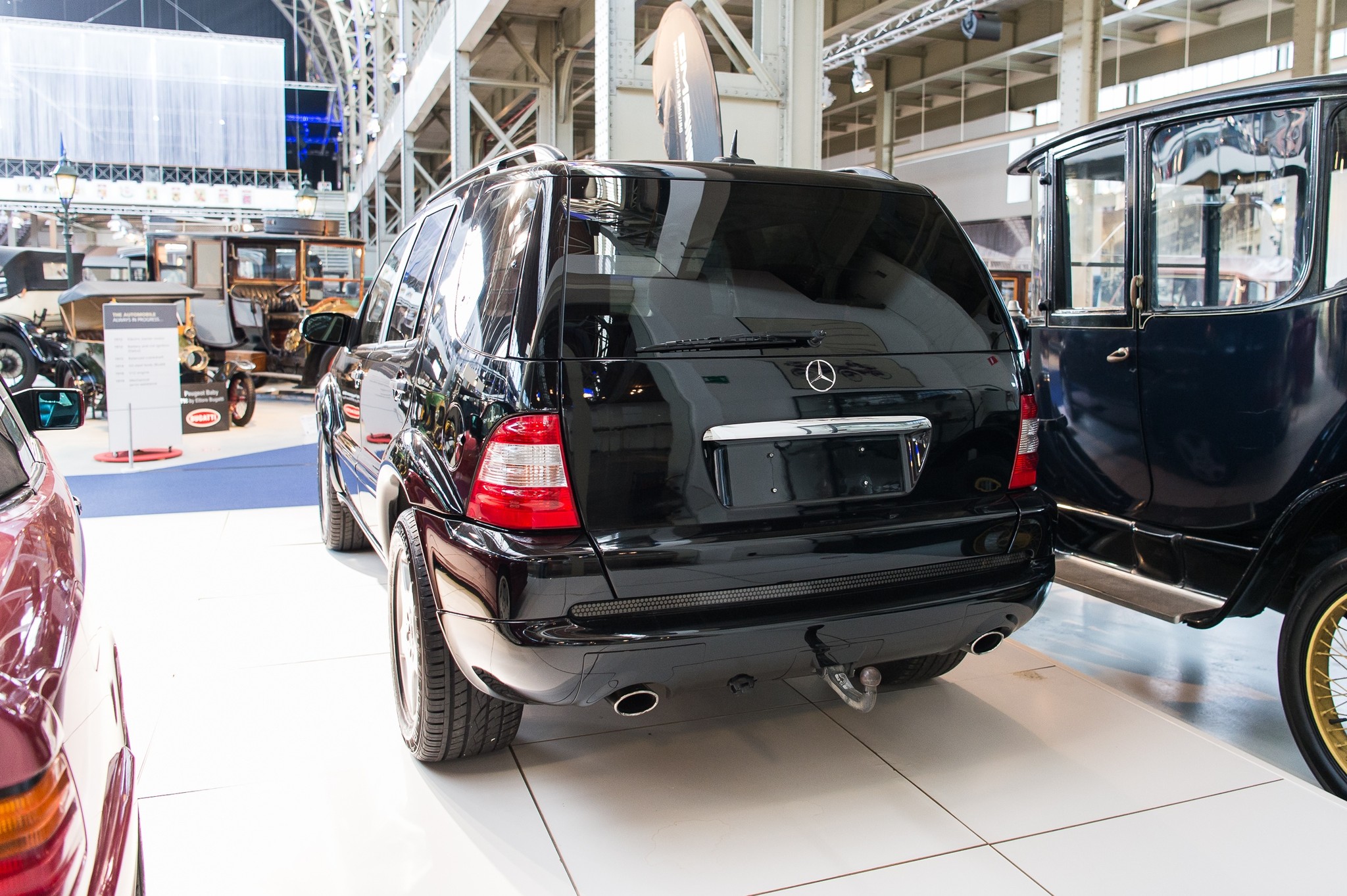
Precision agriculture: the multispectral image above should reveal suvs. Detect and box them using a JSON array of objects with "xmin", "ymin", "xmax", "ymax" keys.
[{"xmin": 295, "ymin": 140, "xmax": 1063, "ymax": 770}]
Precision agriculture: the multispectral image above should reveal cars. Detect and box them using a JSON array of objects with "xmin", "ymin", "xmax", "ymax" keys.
[
  {"xmin": 0, "ymin": 374, "xmax": 151, "ymax": 895},
  {"xmin": 0, "ymin": 213, "xmax": 368, "ymax": 427},
  {"xmin": 1007, "ymin": 69, "xmax": 1347, "ymax": 808}
]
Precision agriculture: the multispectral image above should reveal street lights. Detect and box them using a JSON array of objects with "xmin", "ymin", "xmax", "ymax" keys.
[{"xmin": 47, "ymin": 147, "xmax": 81, "ymax": 289}]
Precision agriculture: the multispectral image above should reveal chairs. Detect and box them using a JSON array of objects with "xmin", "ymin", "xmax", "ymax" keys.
[{"xmin": 642, "ymin": 268, "xmax": 725, "ymax": 339}]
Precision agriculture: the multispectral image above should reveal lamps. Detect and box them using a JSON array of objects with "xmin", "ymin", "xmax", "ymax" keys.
[
  {"xmin": 962, "ymin": 10, "xmax": 1002, "ymax": 42},
  {"xmin": 849, "ymin": 55, "xmax": 874, "ymax": 94},
  {"xmin": 822, "ymin": 76, "xmax": 837, "ymax": 111}
]
